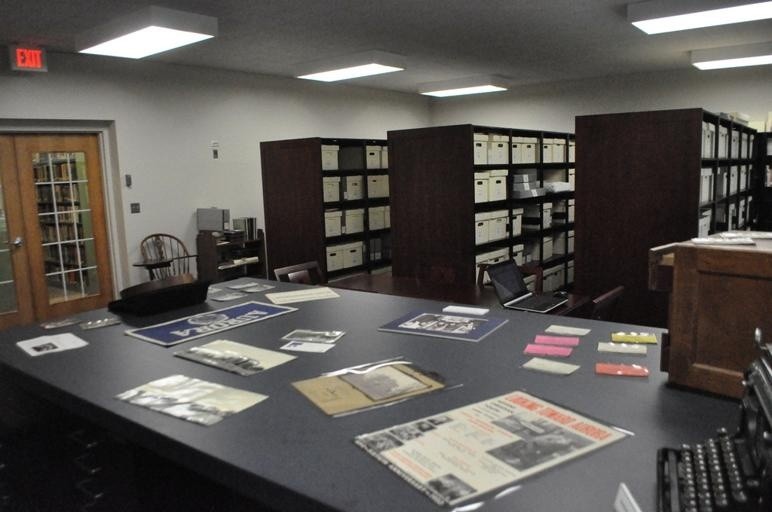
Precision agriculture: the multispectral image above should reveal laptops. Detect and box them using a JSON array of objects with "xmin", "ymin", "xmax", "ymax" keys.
[{"xmin": 485, "ymin": 258, "xmax": 569, "ymax": 313}]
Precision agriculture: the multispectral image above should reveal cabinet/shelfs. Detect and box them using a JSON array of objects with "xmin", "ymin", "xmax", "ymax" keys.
[
  {"xmin": 195, "ymin": 228, "xmax": 268, "ymax": 284},
  {"xmin": 260, "ymin": 136, "xmax": 391, "ymax": 285},
  {"xmin": 574, "ymin": 106, "xmax": 757, "ymax": 327},
  {"xmin": 646, "ymin": 229, "xmax": 771, "ymax": 400},
  {"xmin": 386, "ymin": 122, "xmax": 576, "ymax": 308},
  {"xmin": 750, "ymin": 130, "xmax": 772, "ymax": 234},
  {"xmin": 33, "ymin": 158, "xmax": 89, "ymax": 295}
]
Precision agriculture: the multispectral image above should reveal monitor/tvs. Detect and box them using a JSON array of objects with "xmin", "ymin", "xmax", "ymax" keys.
[
  {"xmin": 196, "ymin": 208, "xmax": 224, "ymax": 239},
  {"xmin": 273, "ymin": 260, "xmax": 325, "ymax": 286}
]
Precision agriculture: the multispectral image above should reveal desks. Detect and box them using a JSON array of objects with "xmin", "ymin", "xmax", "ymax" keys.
[{"xmin": 0, "ymin": 274, "xmax": 741, "ymax": 512}]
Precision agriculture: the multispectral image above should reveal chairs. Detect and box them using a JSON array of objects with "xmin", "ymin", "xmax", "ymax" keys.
[{"xmin": 140, "ymin": 233, "xmax": 190, "ymax": 281}]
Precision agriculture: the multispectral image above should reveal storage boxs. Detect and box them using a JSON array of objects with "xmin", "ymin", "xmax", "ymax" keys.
[
  {"xmin": 696, "ymin": 120, "xmax": 755, "ymax": 238},
  {"xmin": 320, "ymin": 144, "xmax": 389, "ymax": 272},
  {"xmin": 472, "ymin": 131, "xmax": 576, "ymax": 296}
]
